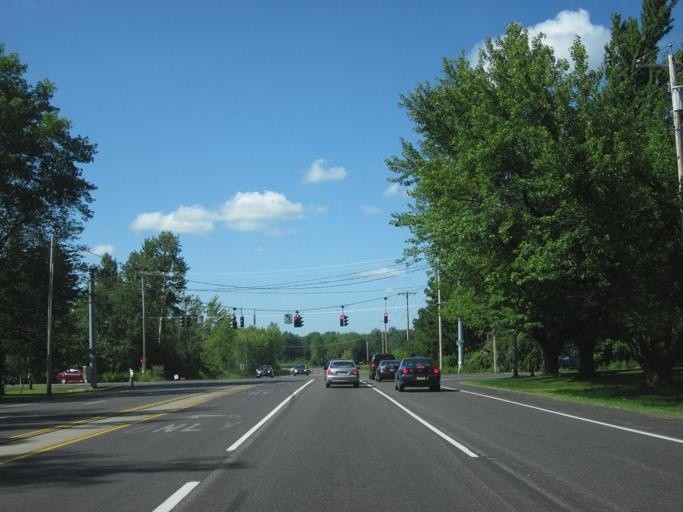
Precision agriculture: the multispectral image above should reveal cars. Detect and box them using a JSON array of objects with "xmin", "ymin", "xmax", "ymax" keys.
[
  {"xmin": 56, "ymin": 367, "xmax": 87, "ymax": 384},
  {"xmin": 292, "ymin": 364, "xmax": 309, "ymax": 376},
  {"xmin": 324, "ymin": 352, "xmax": 440, "ymax": 391},
  {"xmin": 255, "ymin": 364, "xmax": 274, "ymax": 377}
]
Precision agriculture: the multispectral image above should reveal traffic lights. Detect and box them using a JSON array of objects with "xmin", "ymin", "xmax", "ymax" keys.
[
  {"xmin": 239, "ymin": 316, "xmax": 245, "ymax": 329},
  {"xmin": 179, "ymin": 316, "xmax": 191, "ymax": 328},
  {"xmin": 384, "ymin": 312, "xmax": 388, "ymax": 323},
  {"xmin": 340, "ymin": 315, "xmax": 348, "ymax": 327},
  {"xmin": 230, "ymin": 316, "xmax": 237, "ymax": 330},
  {"xmin": 293, "ymin": 317, "xmax": 303, "ymax": 328}
]
